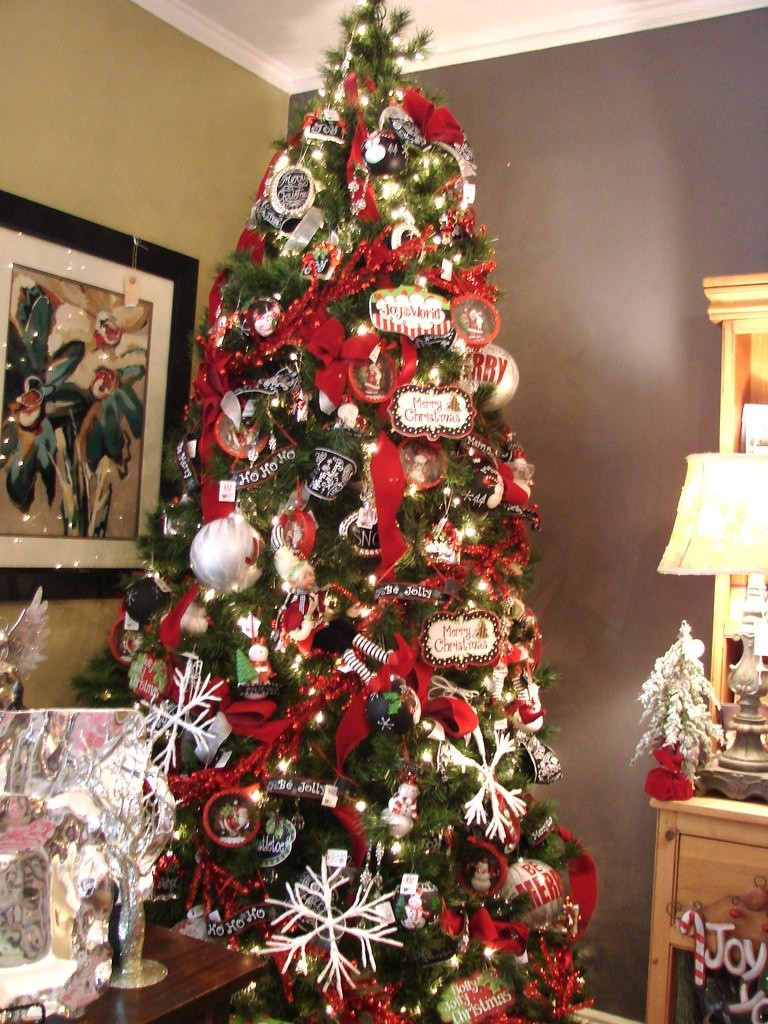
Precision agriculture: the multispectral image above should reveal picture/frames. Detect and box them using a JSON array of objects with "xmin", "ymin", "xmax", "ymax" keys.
[{"xmin": 0, "ymin": 188, "xmax": 201, "ymax": 603}]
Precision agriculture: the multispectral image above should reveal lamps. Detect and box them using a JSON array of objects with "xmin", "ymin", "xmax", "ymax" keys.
[{"xmin": 652, "ymin": 454, "xmax": 768, "ymax": 805}]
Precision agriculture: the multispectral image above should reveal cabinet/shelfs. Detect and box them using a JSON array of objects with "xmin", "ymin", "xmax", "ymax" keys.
[{"xmin": 702, "ymin": 273, "xmax": 768, "ymax": 765}]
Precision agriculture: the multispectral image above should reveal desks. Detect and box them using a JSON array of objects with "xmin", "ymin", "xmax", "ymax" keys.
[
  {"xmin": 37, "ymin": 918, "xmax": 270, "ymax": 1024},
  {"xmin": 643, "ymin": 792, "xmax": 768, "ymax": 1024}
]
